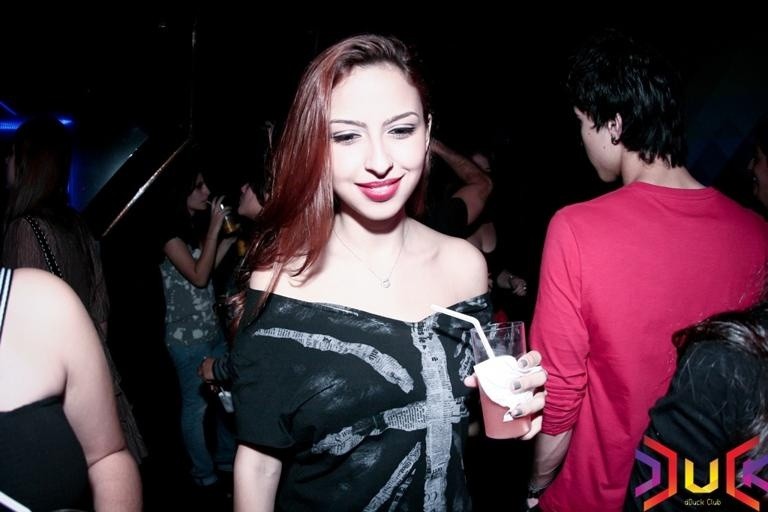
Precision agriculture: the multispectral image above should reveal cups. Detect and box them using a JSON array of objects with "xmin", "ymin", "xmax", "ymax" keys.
[
  {"xmin": 218, "ymin": 204, "xmax": 238, "ymax": 234},
  {"xmin": 470, "ymin": 320, "xmax": 536, "ymax": 440}
]
